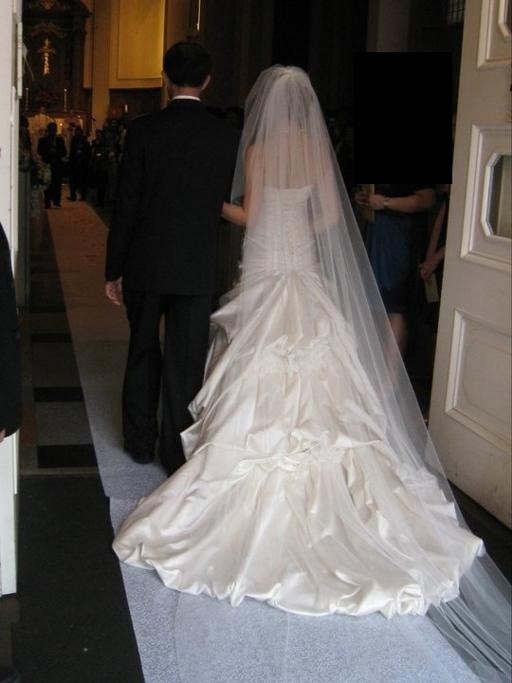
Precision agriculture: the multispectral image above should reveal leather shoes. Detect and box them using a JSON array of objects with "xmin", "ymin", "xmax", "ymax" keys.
[{"xmin": 124, "ymin": 443, "xmax": 178, "ymax": 477}]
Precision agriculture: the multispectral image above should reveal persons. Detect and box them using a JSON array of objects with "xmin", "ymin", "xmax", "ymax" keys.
[
  {"xmin": 35, "ymin": 119, "xmax": 126, "ymax": 209},
  {"xmin": 320, "ymin": 82, "xmax": 452, "ymax": 353},
  {"xmin": 217, "ymin": 64, "xmax": 486, "ymax": 616},
  {"xmin": 102, "ymin": 41, "xmax": 235, "ymax": 477}
]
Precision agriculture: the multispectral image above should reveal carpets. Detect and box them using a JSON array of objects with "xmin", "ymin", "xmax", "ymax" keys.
[{"xmin": 40, "ymin": 194, "xmax": 512, "ymax": 682}]
